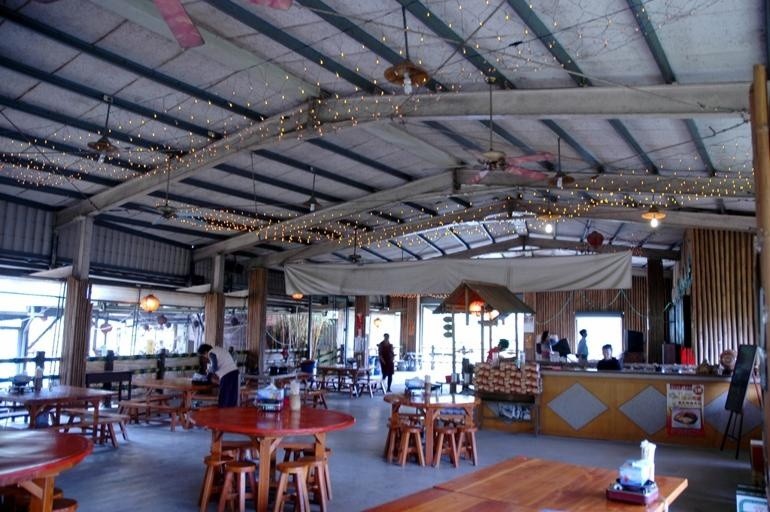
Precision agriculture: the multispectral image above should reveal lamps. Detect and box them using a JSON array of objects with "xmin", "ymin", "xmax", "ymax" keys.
[
  {"xmin": 549, "ymin": 128, "xmax": 574, "ymax": 188},
  {"xmin": 373, "ymin": 310, "xmax": 383, "ymax": 329},
  {"xmin": 640, "ymin": 192, "xmax": 666, "ymax": 229},
  {"xmin": 140, "ymin": 280, "xmax": 160, "ymax": 313},
  {"xmin": 303, "ymin": 167, "xmax": 321, "ymax": 213},
  {"xmin": 469, "ymin": 289, "xmax": 487, "ymax": 318},
  {"xmin": 291, "ymin": 293, "xmax": 304, "ymax": 300},
  {"xmin": 382, "ymin": 0, "xmax": 429, "ymax": 94},
  {"xmin": 87, "ymin": 93, "xmax": 121, "ymax": 164},
  {"xmin": 346, "ymin": 225, "xmax": 362, "ymax": 264},
  {"xmin": 535, "ymin": 189, "xmax": 562, "ymax": 234}
]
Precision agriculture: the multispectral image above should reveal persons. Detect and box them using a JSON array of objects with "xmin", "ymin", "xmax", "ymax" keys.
[
  {"xmin": 540, "ymin": 330, "xmax": 552, "ymax": 360},
  {"xmin": 377, "ymin": 333, "xmax": 395, "ymax": 392},
  {"xmin": 575, "ymin": 329, "xmax": 588, "ymax": 361},
  {"xmin": 354, "ymin": 326, "xmax": 365, "ymax": 369},
  {"xmin": 229, "ymin": 346, "xmax": 239, "ymax": 364},
  {"xmin": 486, "ymin": 339, "xmax": 508, "ymax": 363},
  {"xmin": 197, "ymin": 344, "xmax": 240, "ymax": 408},
  {"xmin": 597, "ymin": 344, "xmax": 621, "ymax": 370}
]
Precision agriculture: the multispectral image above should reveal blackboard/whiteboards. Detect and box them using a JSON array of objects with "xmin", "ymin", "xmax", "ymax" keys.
[{"xmin": 725, "ymin": 344, "xmax": 757, "ymax": 413}]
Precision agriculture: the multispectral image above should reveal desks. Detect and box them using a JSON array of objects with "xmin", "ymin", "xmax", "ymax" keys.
[
  {"xmin": 359, "ymin": 488, "xmax": 566, "ymax": 512},
  {"xmin": 435, "ymin": 453, "xmax": 690, "ymax": 512},
  {"xmin": 1, "ymin": 430, "xmax": 95, "ymax": 512}
]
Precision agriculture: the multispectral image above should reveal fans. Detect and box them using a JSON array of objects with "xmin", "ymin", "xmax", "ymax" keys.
[
  {"xmin": 421, "ymin": 76, "xmax": 556, "ymax": 187},
  {"xmin": 139, "ymin": 146, "xmax": 217, "ymax": 225},
  {"xmin": 144, "ymin": 0, "xmax": 295, "ymax": 51}
]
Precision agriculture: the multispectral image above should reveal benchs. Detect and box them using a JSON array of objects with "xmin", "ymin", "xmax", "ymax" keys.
[
  {"xmin": 239, "ymin": 360, "xmax": 388, "ymax": 410},
  {"xmin": 113, "ymin": 376, "xmax": 219, "ymax": 433},
  {"xmin": 1, "ymin": 385, "xmax": 131, "ymax": 447}
]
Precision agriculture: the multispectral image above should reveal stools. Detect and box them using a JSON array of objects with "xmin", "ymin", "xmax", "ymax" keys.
[
  {"xmin": 53, "ymin": 498, "xmax": 79, "ymax": 511},
  {"xmin": 54, "ymin": 487, "xmax": 63, "ymax": 497},
  {"xmin": 187, "ymin": 389, "xmax": 481, "ymax": 511}
]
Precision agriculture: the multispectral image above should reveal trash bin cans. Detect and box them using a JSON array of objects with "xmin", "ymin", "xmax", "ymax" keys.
[
  {"xmin": 368, "ymin": 356, "xmax": 380, "ymax": 376},
  {"xmin": 300, "ymin": 362, "xmax": 315, "ymax": 382}
]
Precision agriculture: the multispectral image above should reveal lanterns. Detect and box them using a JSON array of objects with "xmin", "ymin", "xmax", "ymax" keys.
[
  {"xmin": 100, "ymin": 323, "xmax": 112, "ymax": 334},
  {"xmin": 469, "ymin": 301, "xmax": 484, "ymax": 313},
  {"xmin": 230, "ymin": 317, "xmax": 240, "ymax": 327},
  {"xmin": 292, "ymin": 293, "xmax": 303, "ymax": 299},
  {"xmin": 137, "ymin": 295, "xmax": 159, "ymax": 313},
  {"xmin": 587, "ymin": 231, "xmax": 604, "ymax": 246},
  {"xmin": 443, "ymin": 324, "xmax": 452, "ymax": 330},
  {"xmin": 443, "ymin": 316, "xmax": 452, "ymax": 322},
  {"xmin": 374, "ymin": 318, "xmax": 381, "ymax": 327},
  {"xmin": 193, "ymin": 320, "xmax": 200, "ymax": 327},
  {"xmin": 443, "ymin": 332, "xmax": 453, "ymax": 337},
  {"xmin": 142, "ymin": 324, "xmax": 150, "ymax": 330},
  {"xmin": 156, "ymin": 315, "xmax": 167, "ymax": 326}
]
